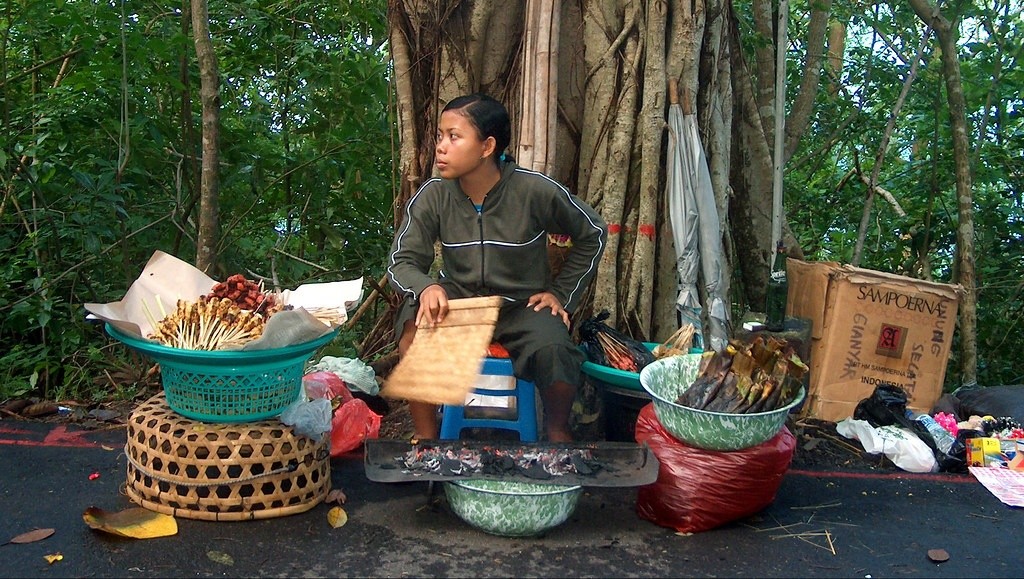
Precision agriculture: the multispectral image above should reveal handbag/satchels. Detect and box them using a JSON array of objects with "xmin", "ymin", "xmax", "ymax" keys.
[{"xmin": 853, "ymin": 384, "xmax": 908, "ymax": 428}]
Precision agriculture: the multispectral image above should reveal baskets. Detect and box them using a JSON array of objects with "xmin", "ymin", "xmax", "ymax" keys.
[{"xmin": 153, "ymin": 350, "xmax": 319, "ymax": 423}]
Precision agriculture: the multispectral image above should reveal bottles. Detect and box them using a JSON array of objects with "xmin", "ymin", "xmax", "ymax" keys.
[{"xmin": 904, "ymin": 409, "xmax": 960, "ymax": 459}]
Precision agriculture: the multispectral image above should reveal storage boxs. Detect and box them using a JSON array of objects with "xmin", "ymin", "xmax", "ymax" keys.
[
  {"xmin": 966, "ymin": 437, "xmax": 1002, "ymax": 466},
  {"xmin": 784, "ymin": 257, "xmax": 968, "ymax": 423}
]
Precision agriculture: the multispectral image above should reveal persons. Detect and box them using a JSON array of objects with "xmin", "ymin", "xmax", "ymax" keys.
[{"xmin": 387, "ymin": 93, "xmax": 608, "ymax": 442}]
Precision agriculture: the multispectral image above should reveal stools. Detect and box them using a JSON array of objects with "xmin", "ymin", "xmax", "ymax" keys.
[{"xmin": 438, "ymin": 358, "xmax": 538, "ymax": 442}]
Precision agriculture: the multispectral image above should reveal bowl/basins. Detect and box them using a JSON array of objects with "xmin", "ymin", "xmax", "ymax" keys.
[
  {"xmin": 639, "ymin": 354, "xmax": 804, "ymax": 451},
  {"xmin": 445, "ymin": 479, "xmax": 584, "ymax": 538}
]
